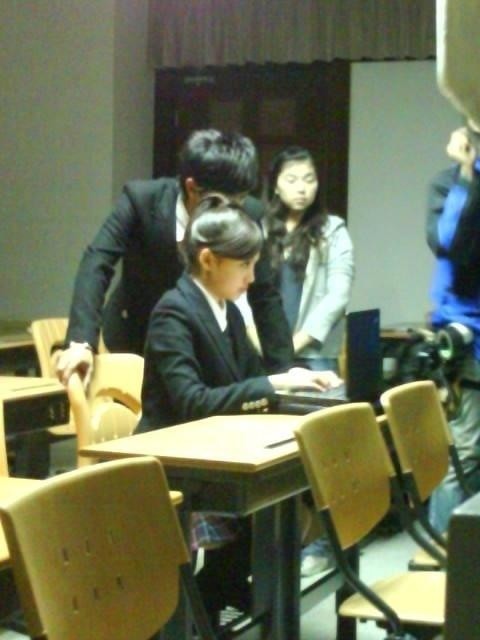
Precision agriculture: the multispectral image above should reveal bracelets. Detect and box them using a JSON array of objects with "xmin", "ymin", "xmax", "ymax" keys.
[{"xmin": 69, "ymin": 339, "xmax": 96, "ymax": 353}]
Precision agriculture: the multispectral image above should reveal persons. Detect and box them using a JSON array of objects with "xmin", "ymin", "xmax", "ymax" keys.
[
  {"xmin": 422, "ymin": 121, "xmax": 480, "ymax": 565},
  {"xmin": 125, "ymin": 197, "xmax": 344, "ymax": 634},
  {"xmin": 50, "ymin": 123, "xmax": 345, "ymax": 395},
  {"xmin": 262, "ymin": 144, "xmax": 357, "ymax": 382}
]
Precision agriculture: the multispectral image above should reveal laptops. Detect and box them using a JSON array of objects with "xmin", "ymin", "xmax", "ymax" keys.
[{"xmin": 274, "ymin": 308, "xmax": 386, "ymax": 406}]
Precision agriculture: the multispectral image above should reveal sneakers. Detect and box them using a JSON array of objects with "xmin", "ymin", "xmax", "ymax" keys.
[
  {"xmin": 197, "ymin": 564, "xmax": 253, "ymax": 612},
  {"xmin": 298, "ymin": 554, "xmax": 339, "ymax": 578}
]
